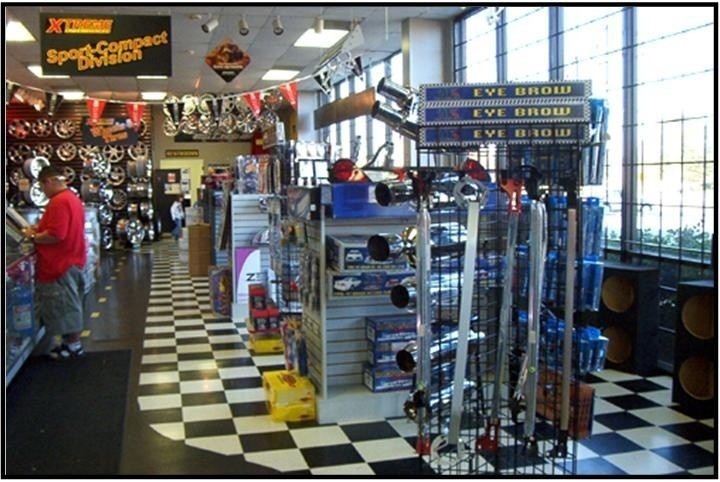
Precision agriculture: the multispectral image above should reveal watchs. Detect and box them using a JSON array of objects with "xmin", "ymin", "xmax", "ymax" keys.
[{"xmin": 30, "ymin": 232, "xmax": 37, "ymax": 243}]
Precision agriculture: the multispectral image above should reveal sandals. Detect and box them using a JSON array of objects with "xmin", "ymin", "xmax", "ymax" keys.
[{"xmin": 46, "ymin": 342, "xmax": 87, "ymax": 362}]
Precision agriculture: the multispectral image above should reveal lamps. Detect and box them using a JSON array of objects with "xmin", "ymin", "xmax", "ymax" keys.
[{"xmin": 194, "ymin": 11, "xmax": 324, "ymax": 35}]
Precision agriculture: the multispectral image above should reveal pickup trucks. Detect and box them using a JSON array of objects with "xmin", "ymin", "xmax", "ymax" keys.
[{"xmin": 598, "ymin": 185, "xmax": 665, "ymax": 214}]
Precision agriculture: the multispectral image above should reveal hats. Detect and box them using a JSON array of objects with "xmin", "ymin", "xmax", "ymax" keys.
[{"xmin": 36, "ymin": 165, "xmax": 68, "ymax": 182}]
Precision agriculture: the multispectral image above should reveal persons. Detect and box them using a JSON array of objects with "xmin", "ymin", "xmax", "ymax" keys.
[
  {"xmin": 20, "ymin": 165, "xmax": 87, "ymax": 361},
  {"xmin": 170, "ymin": 194, "xmax": 184, "ymax": 240}
]
